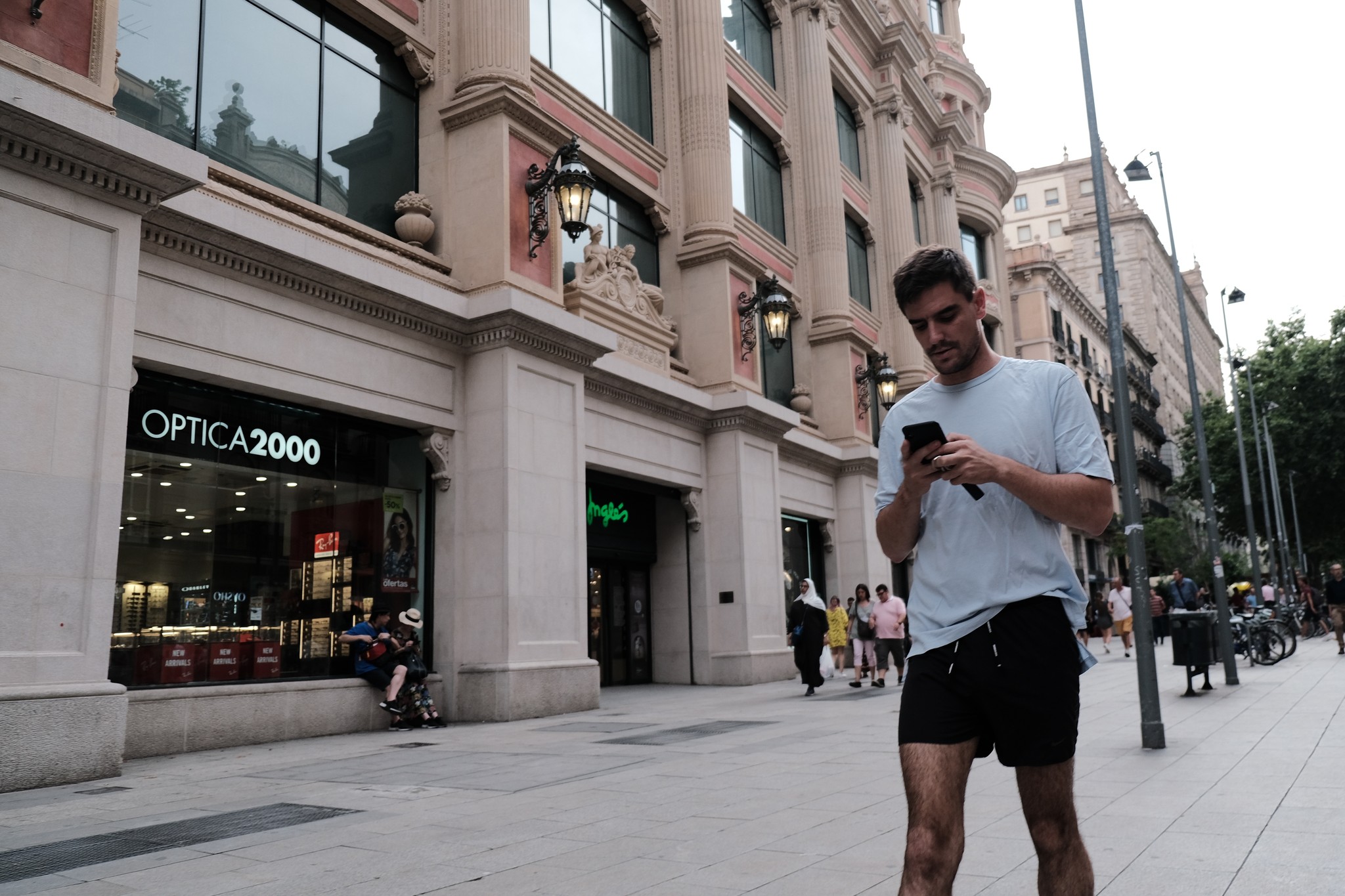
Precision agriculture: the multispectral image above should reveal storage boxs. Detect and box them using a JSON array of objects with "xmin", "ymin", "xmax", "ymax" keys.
[
  {"xmin": 196, "ymin": 641, "xmax": 242, "ymax": 681},
  {"xmin": 242, "ymin": 640, "xmax": 282, "ymax": 679},
  {"xmin": 132, "ymin": 641, "xmax": 195, "ymax": 683}
]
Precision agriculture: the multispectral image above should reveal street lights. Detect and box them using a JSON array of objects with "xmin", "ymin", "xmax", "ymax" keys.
[
  {"xmin": 1223, "ymin": 354, "xmax": 1295, "ymax": 637},
  {"xmin": 1218, "ymin": 280, "xmax": 1274, "ymax": 664},
  {"xmin": 1124, "ymin": 150, "xmax": 1243, "ymax": 685}
]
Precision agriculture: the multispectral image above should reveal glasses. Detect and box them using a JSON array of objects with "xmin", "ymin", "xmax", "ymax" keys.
[
  {"xmin": 877, "ymin": 590, "xmax": 886, "ymax": 596},
  {"xmin": 848, "ymin": 602, "xmax": 852, "ymax": 605},
  {"xmin": 391, "ymin": 521, "xmax": 407, "ymax": 531},
  {"xmin": 125, "ymin": 592, "xmax": 151, "ymax": 631},
  {"xmin": 799, "ymin": 585, "xmax": 808, "ymax": 588}
]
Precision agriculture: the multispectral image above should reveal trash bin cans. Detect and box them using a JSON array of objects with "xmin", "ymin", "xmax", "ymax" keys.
[{"xmin": 1168, "ymin": 611, "xmax": 1224, "ymax": 666}]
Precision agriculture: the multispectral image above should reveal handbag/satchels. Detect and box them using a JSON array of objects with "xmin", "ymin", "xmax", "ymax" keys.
[
  {"xmin": 819, "ymin": 643, "xmax": 834, "ymax": 678},
  {"xmin": 364, "ymin": 635, "xmax": 391, "ymax": 666},
  {"xmin": 856, "ymin": 617, "xmax": 875, "ymax": 640},
  {"xmin": 792, "ymin": 625, "xmax": 803, "ymax": 635},
  {"xmin": 1099, "ymin": 616, "xmax": 1113, "ymax": 629},
  {"xmin": 1184, "ymin": 601, "xmax": 1197, "ymax": 611}
]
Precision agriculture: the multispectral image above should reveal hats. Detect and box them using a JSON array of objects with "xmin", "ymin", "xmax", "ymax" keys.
[{"xmin": 399, "ymin": 608, "xmax": 423, "ymax": 629}]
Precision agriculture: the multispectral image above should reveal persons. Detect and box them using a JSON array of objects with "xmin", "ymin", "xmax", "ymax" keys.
[
  {"xmin": 1168, "ymin": 567, "xmax": 1210, "ymax": 612},
  {"xmin": 388, "ymin": 608, "xmax": 448, "ymax": 729},
  {"xmin": 382, "ymin": 507, "xmax": 419, "ymax": 589},
  {"xmin": 845, "ymin": 583, "xmax": 912, "ymax": 688},
  {"xmin": 1325, "ymin": 563, "xmax": 1345, "ymax": 655},
  {"xmin": 1226, "ymin": 587, "xmax": 1259, "ymax": 613},
  {"xmin": 337, "ymin": 604, "xmax": 414, "ymax": 732},
  {"xmin": 826, "ymin": 595, "xmax": 849, "ymax": 679},
  {"xmin": 1277, "ymin": 587, "xmax": 1287, "ymax": 607},
  {"xmin": 1261, "ymin": 581, "xmax": 1276, "ymax": 609},
  {"xmin": 873, "ymin": 242, "xmax": 1117, "ymax": 896},
  {"xmin": 1295, "ymin": 578, "xmax": 1330, "ymax": 640},
  {"xmin": 1149, "ymin": 589, "xmax": 1166, "ymax": 646},
  {"xmin": 1076, "ymin": 575, "xmax": 1135, "ymax": 657},
  {"xmin": 784, "ymin": 578, "xmax": 832, "ymax": 696}
]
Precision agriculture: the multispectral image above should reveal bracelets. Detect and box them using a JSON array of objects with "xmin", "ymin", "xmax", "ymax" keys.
[{"xmin": 389, "ymin": 636, "xmax": 393, "ymax": 639}]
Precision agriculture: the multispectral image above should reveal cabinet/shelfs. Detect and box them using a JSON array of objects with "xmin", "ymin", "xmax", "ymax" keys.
[
  {"xmin": 305, "ymin": 556, "xmax": 356, "ymax": 614},
  {"xmin": 279, "ymin": 617, "xmax": 356, "ymax": 660}
]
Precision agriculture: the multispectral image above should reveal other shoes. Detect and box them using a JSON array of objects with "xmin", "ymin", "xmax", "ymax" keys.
[
  {"xmin": 1103, "ymin": 644, "xmax": 1110, "ymax": 653},
  {"xmin": 872, "ymin": 678, "xmax": 884, "ymax": 688},
  {"xmin": 839, "ymin": 673, "xmax": 846, "ymax": 678},
  {"xmin": 849, "ymin": 681, "xmax": 861, "ymax": 687},
  {"xmin": 898, "ymin": 681, "xmax": 903, "ymax": 686},
  {"xmin": 1338, "ymin": 650, "xmax": 1344, "ymax": 654},
  {"xmin": 805, "ymin": 687, "xmax": 814, "ymax": 696},
  {"xmin": 862, "ymin": 674, "xmax": 868, "ymax": 678},
  {"xmin": 1125, "ymin": 653, "xmax": 1129, "ymax": 657}
]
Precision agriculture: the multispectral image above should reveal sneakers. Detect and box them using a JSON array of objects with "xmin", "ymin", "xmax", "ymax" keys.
[
  {"xmin": 432, "ymin": 716, "xmax": 447, "ymax": 727},
  {"xmin": 388, "ymin": 719, "xmax": 412, "ymax": 731},
  {"xmin": 421, "ymin": 716, "xmax": 439, "ymax": 729},
  {"xmin": 378, "ymin": 698, "xmax": 402, "ymax": 715}
]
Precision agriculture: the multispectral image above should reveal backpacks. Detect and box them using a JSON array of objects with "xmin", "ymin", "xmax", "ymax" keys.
[
  {"xmin": 399, "ymin": 646, "xmax": 428, "ymax": 683},
  {"xmin": 1304, "ymin": 587, "xmax": 1325, "ymax": 606}
]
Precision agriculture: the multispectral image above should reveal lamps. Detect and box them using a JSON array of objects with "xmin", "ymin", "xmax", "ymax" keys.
[
  {"xmin": 735, "ymin": 273, "xmax": 796, "ymax": 364},
  {"xmin": 854, "ymin": 351, "xmax": 900, "ymax": 421},
  {"xmin": 520, "ymin": 128, "xmax": 606, "ymax": 266}
]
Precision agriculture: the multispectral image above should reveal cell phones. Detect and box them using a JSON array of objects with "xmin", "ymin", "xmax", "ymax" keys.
[{"xmin": 902, "ymin": 421, "xmax": 948, "ymax": 468}]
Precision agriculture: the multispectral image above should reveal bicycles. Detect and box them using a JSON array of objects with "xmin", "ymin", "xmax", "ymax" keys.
[{"xmin": 1218, "ymin": 596, "xmax": 1336, "ymax": 668}]
